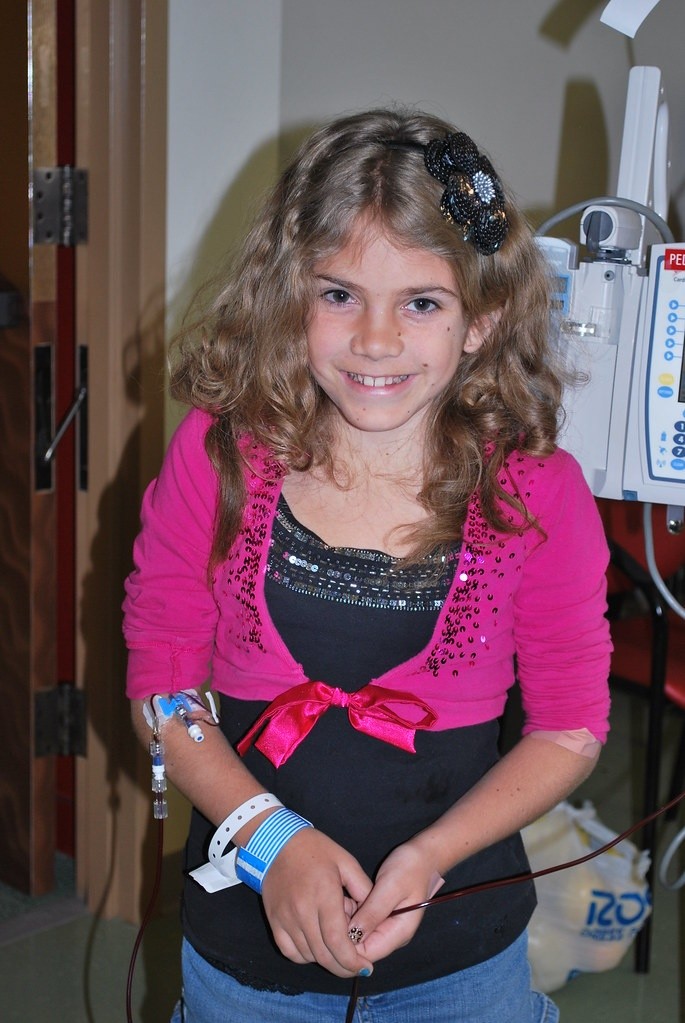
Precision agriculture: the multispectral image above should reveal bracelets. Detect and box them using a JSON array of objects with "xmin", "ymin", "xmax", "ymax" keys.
[
  {"xmin": 236, "ymin": 808, "xmax": 315, "ymax": 895},
  {"xmin": 188, "ymin": 792, "xmax": 285, "ymax": 893}
]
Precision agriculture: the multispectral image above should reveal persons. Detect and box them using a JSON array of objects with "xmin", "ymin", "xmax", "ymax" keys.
[{"xmin": 120, "ymin": 108, "xmax": 615, "ymax": 1023}]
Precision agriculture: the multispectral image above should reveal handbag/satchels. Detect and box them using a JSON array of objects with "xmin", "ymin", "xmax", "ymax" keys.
[{"xmin": 517, "ymin": 799, "xmax": 655, "ymax": 994}]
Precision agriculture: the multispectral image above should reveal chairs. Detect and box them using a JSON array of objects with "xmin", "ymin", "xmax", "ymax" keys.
[{"xmin": 503, "ymin": 496, "xmax": 685, "ymax": 974}]
